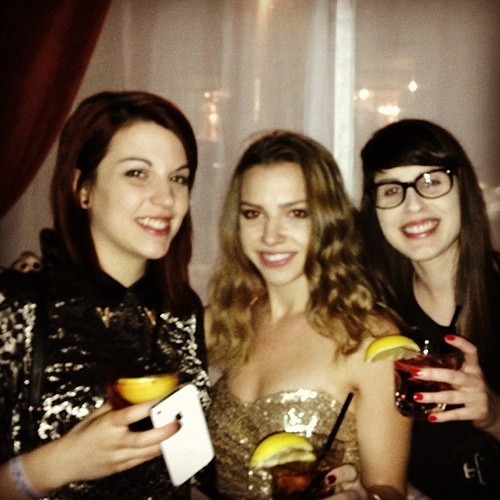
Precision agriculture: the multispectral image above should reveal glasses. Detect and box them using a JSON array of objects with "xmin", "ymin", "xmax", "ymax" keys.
[{"xmin": 368, "ymin": 168, "xmax": 456, "ymax": 209}]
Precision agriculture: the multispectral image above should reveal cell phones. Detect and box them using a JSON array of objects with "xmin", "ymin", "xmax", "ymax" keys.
[{"xmin": 151, "ymin": 384, "xmax": 215, "ymax": 488}]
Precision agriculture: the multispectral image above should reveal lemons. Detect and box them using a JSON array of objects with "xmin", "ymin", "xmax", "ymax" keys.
[
  {"xmin": 364, "ymin": 336, "xmax": 421, "ymax": 363},
  {"xmin": 250, "ymin": 432, "xmax": 316, "ymax": 468},
  {"xmin": 114, "ymin": 374, "xmax": 179, "ymax": 404}
]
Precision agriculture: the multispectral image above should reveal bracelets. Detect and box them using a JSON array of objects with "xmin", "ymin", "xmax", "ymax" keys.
[{"xmin": 8, "ymin": 454, "xmax": 40, "ymax": 500}]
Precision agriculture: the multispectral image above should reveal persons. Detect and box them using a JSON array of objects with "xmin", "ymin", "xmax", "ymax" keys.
[
  {"xmin": 11, "ymin": 126, "xmax": 413, "ymax": 500},
  {"xmin": 0, "ymin": 91, "xmax": 211, "ymax": 500},
  {"xmin": 357, "ymin": 118, "xmax": 500, "ymax": 500}
]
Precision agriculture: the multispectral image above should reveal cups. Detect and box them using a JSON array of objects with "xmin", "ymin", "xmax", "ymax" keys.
[
  {"xmin": 393, "ymin": 345, "xmax": 460, "ymax": 419},
  {"xmin": 269, "ymin": 431, "xmax": 347, "ymax": 500},
  {"xmin": 105, "ymin": 357, "xmax": 180, "ymax": 431}
]
